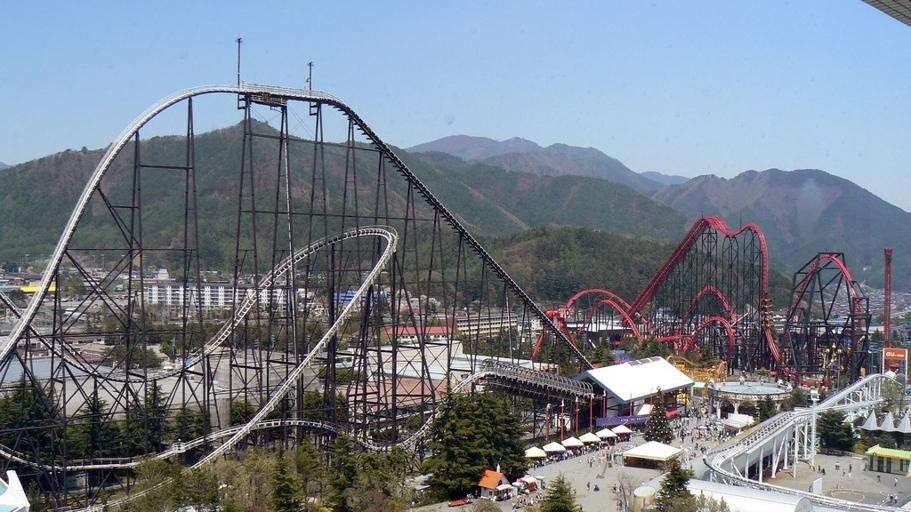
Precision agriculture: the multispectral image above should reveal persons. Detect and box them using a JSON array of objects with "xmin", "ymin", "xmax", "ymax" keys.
[
  {"xmin": 891, "ymin": 494, "xmax": 893, "ymax": 501},
  {"xmin": 672, "ymin": 401, "xmax": 737, "ymax": 460},
  {"xmin": 895, "ymin": 494, "xmax": 898, "ymax": 502},
  {"xmin": 529, "ymin": 432, "xmax": 632, "ymax": 468},
  {"xmin": 823, "ymin": 468, "xmax": 826, "ymax": 475},
  {"xmin": 896, "ymin": 477, "xmax": 899, "ymax": 486},
  {"xmin": 593, "ymin": 484, "xmax": 600, "ymax": 493},
  {"xmin": 818, "ymin": 463, "xmax": 821, "ymax": 473},
  {"xmin": 492, "ymin": 487, "xmax": 544, "ymax": 511},
  {"xmin": 850, "ymin": 463, "xmax": 853, "ymax": 471},
  {"xmin": 877, "ymin": 473, "xmax": 881, "ymax": 481},
  {"xmin": 587, "ymin": 480, "xmax": 591, "ymax": 495}
]
